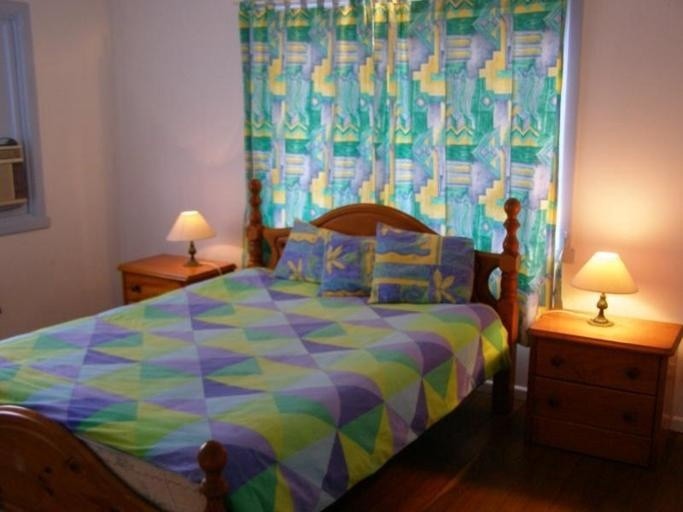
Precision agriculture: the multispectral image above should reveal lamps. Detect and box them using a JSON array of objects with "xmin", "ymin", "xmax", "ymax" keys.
[
  {"xmin": 567, "ymin": 249, "xmax": 641, "ymax": 328},
  {"xmin": 163, "ymin": 210, "xmax": 218, "ymax": 266}
]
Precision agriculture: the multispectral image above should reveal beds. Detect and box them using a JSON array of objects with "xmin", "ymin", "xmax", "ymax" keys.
[{"xmin": 0, "ymin": 176, "xmax": 521, "ymax": 512}]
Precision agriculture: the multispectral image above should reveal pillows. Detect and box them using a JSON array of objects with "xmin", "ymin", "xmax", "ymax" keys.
[
  {"xmin": 364, "ymin": 220, "xmax": 475, "ymax": 305},
  {"xmin": 314, "ymin": 229, "xmax": 376, "ymax": 299},
  {"xmin": 268, "ymin": 217, "xmax": 340, "ymax": 285}
]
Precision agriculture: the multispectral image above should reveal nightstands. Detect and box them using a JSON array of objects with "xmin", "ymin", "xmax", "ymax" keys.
[
  {"xmin": 524, "ymin": 307, "xmax": 682, "ymax": 468},
  {"xmin": 116, "ymin": 253, "xmax": 237, "ymax": 305}
]
